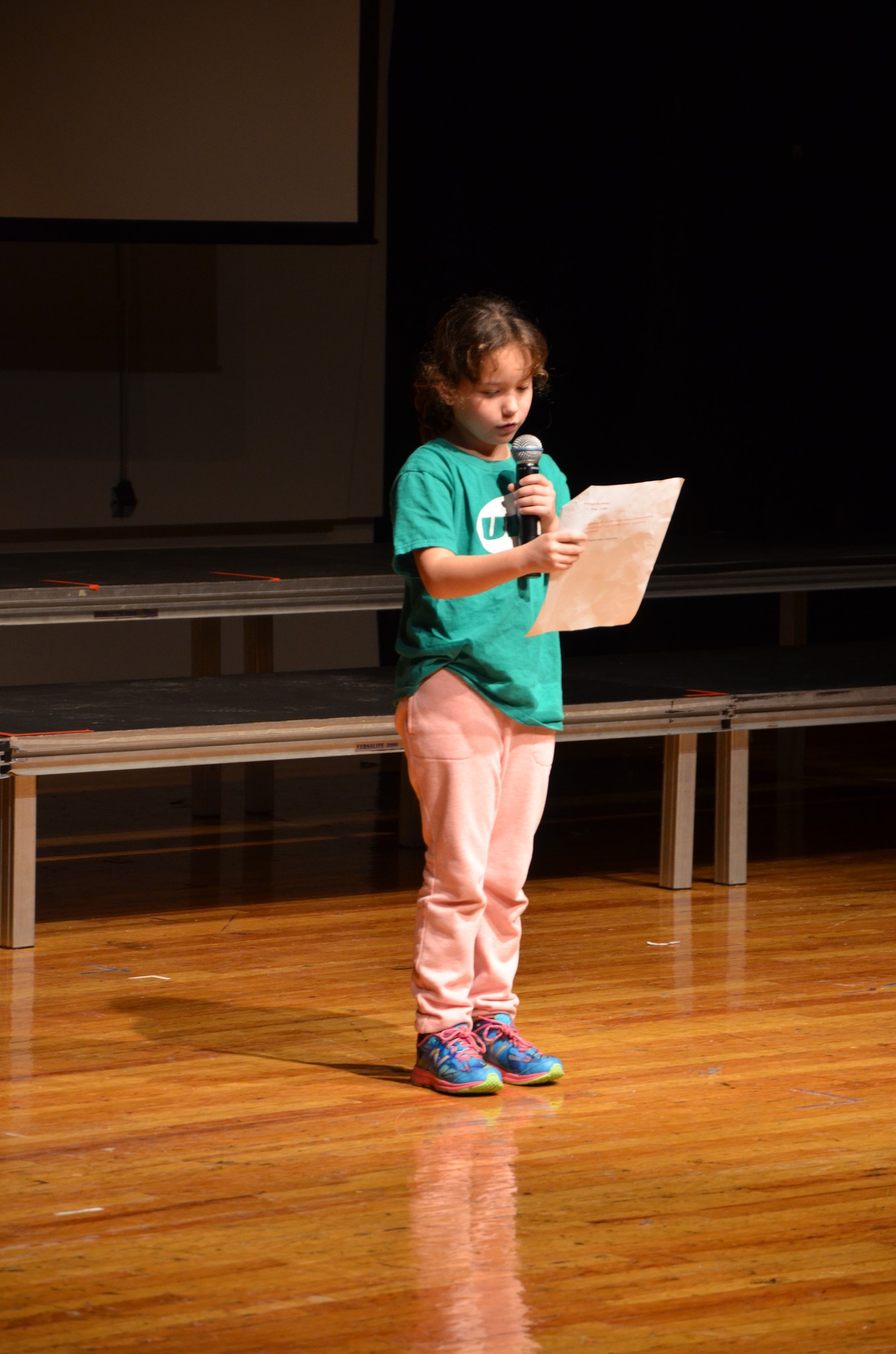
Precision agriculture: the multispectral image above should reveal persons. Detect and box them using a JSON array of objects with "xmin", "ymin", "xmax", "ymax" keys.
[{"xmin": 381, "ymin": 296, "xmax": 571, "ymax": 1096}]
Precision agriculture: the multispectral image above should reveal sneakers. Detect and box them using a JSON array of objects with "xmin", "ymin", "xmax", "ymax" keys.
[
  {"xmin": 409, "ymin": 1023, "xmax": 503, "ymax": 1095},
  {"xmin": 473, "ymin": 1013, "xmax": 564, "ymax": 1083}
]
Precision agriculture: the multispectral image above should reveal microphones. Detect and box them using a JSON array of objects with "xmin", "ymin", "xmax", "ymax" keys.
[{"xmin": 509, "ymin": 434, "xmax": 545, "ymax": 580}]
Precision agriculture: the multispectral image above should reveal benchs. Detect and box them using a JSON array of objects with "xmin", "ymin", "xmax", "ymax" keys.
[{"xmin": 0, "ymin": 656, "xmax": 896, "ymax": 947}]
